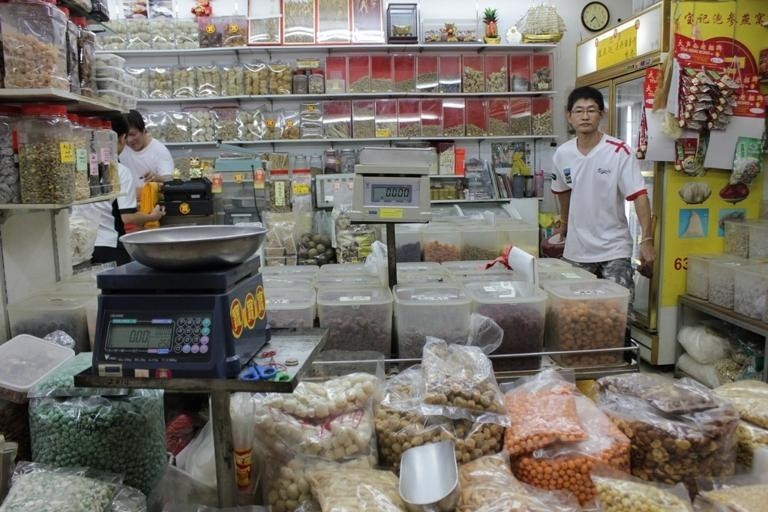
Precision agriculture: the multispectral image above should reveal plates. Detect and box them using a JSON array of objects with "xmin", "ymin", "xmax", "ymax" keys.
[{"xmin": 117, "ymin": 224, "xmax": 270, "ymax": 269}]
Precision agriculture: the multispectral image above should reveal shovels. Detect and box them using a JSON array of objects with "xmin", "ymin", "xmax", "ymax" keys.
[{"xmin": 398, "ymin": 439, "xmax": 461, "ymax": 512}]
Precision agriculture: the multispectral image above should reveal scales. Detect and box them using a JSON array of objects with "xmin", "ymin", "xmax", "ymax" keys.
[
  {"xmin": 91, "ymin": 254, "xmax": 272, "ymax": 379},
  {"xmin": 349, "ymin": 164, "xmax": 433, "ymax": 292}
]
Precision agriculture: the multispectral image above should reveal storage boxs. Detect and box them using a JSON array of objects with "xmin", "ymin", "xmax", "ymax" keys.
[
  {"xmin": 0, "ymin": 278, "xmax": 101, "ymax": 406},
  {"xmin": 687, "ymin": 211, "xmax": 767, "ymax": 324}
]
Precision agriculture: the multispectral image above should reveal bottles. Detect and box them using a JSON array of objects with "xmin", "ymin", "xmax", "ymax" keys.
[
  {"xmin": 293, "ymin": 67, "xmax": 324, "ymax": 94},
  {"xmin": 512, "ymin": 169, "xmax": 545, "ymax": 197}
]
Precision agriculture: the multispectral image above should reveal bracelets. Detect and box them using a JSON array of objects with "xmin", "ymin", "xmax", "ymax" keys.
[
  {"xmin": 638, "ymin": 236, "xmax": 652, "ymax": 246},
  {"xmin": 558, "ymin": 218, "xmax": 568, "ymax": 226}
]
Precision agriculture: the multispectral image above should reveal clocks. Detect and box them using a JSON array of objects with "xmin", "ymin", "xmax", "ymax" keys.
[{"xmin": 580, "ymin": 0, "xmax": 610, "ymax": 32}]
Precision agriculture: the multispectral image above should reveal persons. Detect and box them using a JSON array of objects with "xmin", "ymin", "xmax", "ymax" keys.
[
  {"xmin": 70, "ymin": 110, "xmax": 166, "ymax": 265},
  {"xmin": 120, "ymin": 111, "xmax": 174, "ymax": 187},
  {"xmin": 550, "ymin": 86, "xmax": 655, "ymax": 338}
]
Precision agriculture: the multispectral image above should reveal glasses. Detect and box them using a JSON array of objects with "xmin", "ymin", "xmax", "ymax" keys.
[{"xmin": 570, "ymin": 109, "xmax": 601, "ymax": 116}]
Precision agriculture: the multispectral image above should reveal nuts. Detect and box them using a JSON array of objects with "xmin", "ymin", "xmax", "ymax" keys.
[{"xmin": 0, "ymin": 221, "xmax": 767, "ymax": 512}]
